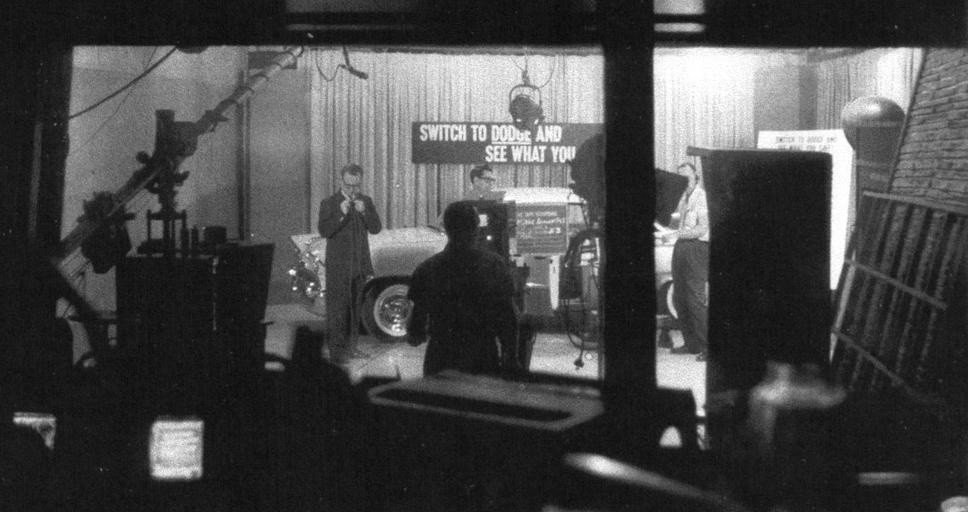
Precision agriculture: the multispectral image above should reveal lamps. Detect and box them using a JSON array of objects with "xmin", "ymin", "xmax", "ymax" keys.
[{"xmin": 505, "ymin": 50, "xmax": 551, "ymax": 140}]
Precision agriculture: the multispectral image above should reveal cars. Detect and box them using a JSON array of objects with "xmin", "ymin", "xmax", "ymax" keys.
[{"xmin": 288, "ymin": 186, "xmax": 680, "ymax": 343}]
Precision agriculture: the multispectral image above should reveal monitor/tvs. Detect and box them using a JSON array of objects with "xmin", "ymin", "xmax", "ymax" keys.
[
  {"xmin": 10, "ymin": 410, "xmax": 60, "ymax": 458},
  {"xmin": 147, "ymin": 414, "xmax": 210, "ymax": 484}
]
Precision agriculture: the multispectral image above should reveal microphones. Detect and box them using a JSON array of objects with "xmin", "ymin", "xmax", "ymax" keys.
[{"xmin": 341, "ymin": 64, "xmax": 369, "ymax": 81}]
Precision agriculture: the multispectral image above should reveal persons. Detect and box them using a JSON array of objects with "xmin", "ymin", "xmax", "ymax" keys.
[
  {"xmin": 317, "ymin": 162, "xmax": 383, "ymax": 366},
  {"xmin": 404, "ymin": 199, "xmax": 525, "ymax": 377},
  {"xmin": 461, "ymin": 163, "xmax": 496, "ymax": 199},
  {"xmin": 662, "ymin": 161, "xmax": 709, "ymax": 362}
]
